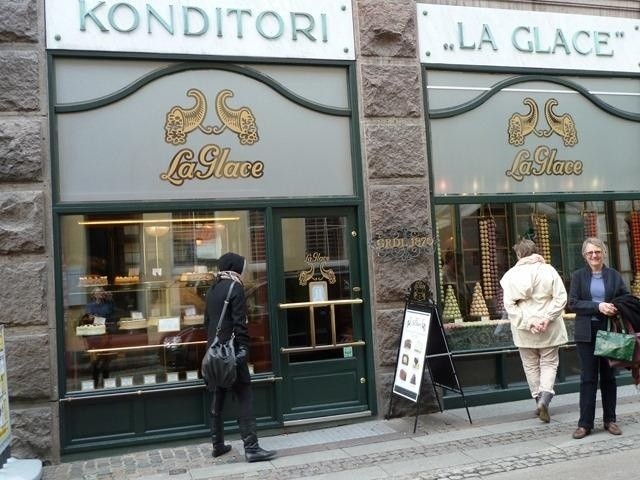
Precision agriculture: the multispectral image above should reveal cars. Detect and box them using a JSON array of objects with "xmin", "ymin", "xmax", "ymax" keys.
[{"xmin": 159, "ymin": 260, "xmax": 354, "ymax": 370}]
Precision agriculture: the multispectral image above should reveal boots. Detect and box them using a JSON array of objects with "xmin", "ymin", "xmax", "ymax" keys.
[
  {"xmin": 537, "ymin": 389, "xmax": 554, "ymax": 424},
  {"xmin": 534, "ymin": 392, "xmax": 542, "ymax": 417}
]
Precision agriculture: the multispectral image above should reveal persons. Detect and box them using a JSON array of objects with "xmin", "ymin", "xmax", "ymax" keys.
[
  {"xmin": 203, "ymin": 252, "xmax": 279, "ymax": 463},
  {"xmin": 566, "ymin": 235, "xmax": 629, "ymax": 440},
  {"xmin": 81, "ymin": 285, "xmax": 117, "ymax": 390},
  {"xmin": 498, "ymin": 236, "xmax": 568, "ymax": 425},
  {"xmin": 442, "ymin": 251, "xmax": 470, "ymax": 314}
]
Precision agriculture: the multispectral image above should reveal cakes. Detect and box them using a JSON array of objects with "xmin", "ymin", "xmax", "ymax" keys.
[{"xmin": 75, "ymin": 272, "xmax": 216, "ymax": 334}]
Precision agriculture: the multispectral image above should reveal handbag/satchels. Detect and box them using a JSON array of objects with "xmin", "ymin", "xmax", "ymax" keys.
[
  {"xmin": 608, "ymin": 314, "xmax": 640, "ymax": 371},
  {"xmin": 197, "ymin": 334, "xmax": 238, "ymax": 394},
  {"xmin": 592, "ymin": 310, "xmax": 635, "ymax": 363}
]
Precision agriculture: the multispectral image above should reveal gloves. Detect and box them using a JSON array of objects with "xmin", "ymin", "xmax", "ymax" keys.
[{"xmin": 235, "ymin": 344, "xmax": 251, "ymax": 366}]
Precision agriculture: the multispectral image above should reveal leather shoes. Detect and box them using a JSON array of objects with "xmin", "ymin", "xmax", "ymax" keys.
[
  {"xmin": 211, "ymin": 444, "xmax": 233, "ymax": 457},
  {"xmin": 572, "ymin": 426, "xmax": 592, "ymax": 440},
  {"xmin": 245, "ymin": 446, "xmax": 278, "ymax": 464},
  {"xmin": 603, "ymin": 420, "xmax": 622, "ymax": 437}
]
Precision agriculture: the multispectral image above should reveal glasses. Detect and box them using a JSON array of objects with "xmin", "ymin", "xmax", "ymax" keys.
[{"xmin": 585, "ymin": 250, "xmax": 604, "ymax": 255}]
